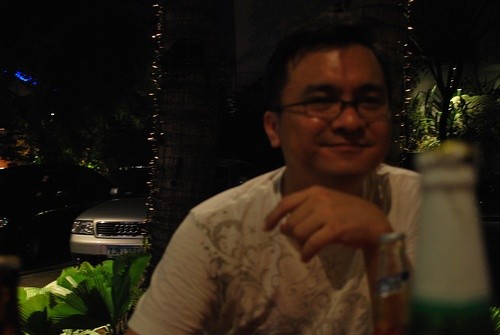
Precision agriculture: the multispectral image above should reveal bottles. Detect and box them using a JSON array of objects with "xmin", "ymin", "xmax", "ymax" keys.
[
  {"xmin": 409, "ymin": 139, "xmax": 490, "ymax": 335},
  {"xmin": 371, "ymin": 231, "xmax": 414, "ymax": 335}
]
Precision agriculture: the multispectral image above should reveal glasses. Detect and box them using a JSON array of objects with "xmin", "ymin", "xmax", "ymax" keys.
[{"xmin": 281, "ymin": 95, "xmax": 391, "ymax": 118}]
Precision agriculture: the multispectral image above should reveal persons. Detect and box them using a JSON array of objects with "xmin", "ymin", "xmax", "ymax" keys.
[{"xmin": 124, "ymin": 16, "xmax": 421, "ymax": 335}]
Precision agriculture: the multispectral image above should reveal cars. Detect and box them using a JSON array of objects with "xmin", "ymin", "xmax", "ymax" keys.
[
  {"xmin": 69, "ymin": 176, "xmax": 150, "ymax": 267},
  {"xmin": 1, "ymin": 165, "xmax": 125, "ymax": 272}
]
